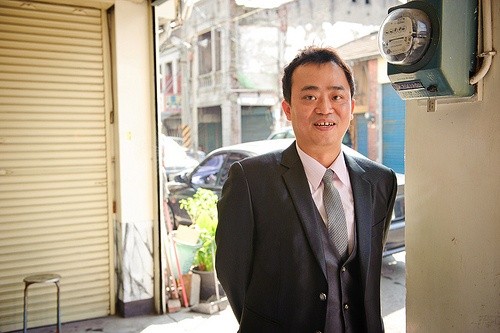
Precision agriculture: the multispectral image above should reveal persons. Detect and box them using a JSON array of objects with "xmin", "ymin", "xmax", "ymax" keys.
[{"xmin": 215, "ymin": 45, "xmax": 399, "ymax": 333}]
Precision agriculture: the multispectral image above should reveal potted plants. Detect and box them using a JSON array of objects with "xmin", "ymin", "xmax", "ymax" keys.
[{"xmin": 178, "ymin": 188, "xmax": 225, "ymax": 300}]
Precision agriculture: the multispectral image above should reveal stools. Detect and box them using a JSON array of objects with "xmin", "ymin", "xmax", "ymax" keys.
[{"xmin": 22, "ymin": 273, "xmax": 62, "ymax": 333}]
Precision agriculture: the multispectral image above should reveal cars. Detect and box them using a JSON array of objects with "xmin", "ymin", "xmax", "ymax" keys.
[
  {"xmin": 162, "ymin": 132, "xmax": 199, "ymax": 182},
  {"xmin": 163, "ymin": 135, "xmax": 405, "ymax": 261}
]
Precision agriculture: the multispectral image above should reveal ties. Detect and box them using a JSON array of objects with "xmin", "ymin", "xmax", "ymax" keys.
[{"xmin": 322, "ymin": 169, "xmax": 349, "ymax": 260}]
